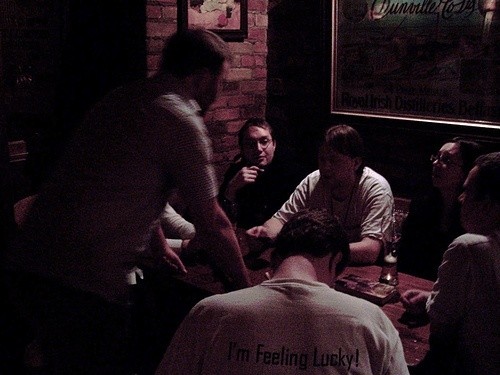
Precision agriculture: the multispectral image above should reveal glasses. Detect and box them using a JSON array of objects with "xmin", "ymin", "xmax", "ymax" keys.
[{"xmin": 430, "ymin": 154, "xmax": 460, "ymax": 168}]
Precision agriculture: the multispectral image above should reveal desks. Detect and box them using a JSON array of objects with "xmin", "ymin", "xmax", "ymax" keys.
[{"xmin": 155, "ymin": 227, "xmax": 458, "ymax": 375}]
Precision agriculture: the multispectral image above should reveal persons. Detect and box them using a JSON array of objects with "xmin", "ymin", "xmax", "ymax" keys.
[
  {"xmin": 216, "ymin": 119, "xmax": 299, "ymax": 229},
  {"xmin": 400, "ymin": 136, "xmax": 500, "ymax": 375},
  {"xmin": 157, "ymin": 201, "xmax": 237, "ymax": 257},
  {"xmin": 246, "ymin": 124, "xmax": 396, "ymax": 265},
  {"xmin": 154, "ymin": 206, "xmax": 410, "ymax": 375},
  {"xmin": 15, "ymin": 27, "xmax": 264, "ymax": 375}
]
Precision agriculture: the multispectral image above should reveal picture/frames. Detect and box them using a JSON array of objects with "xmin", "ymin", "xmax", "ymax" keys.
[
  {"xmin": 331, "ymin": 0, "xmax": 500, "ymax": 130},
  {"xmin": 179, "ymin": 0, "xmax": 248, "ymax": 38}
]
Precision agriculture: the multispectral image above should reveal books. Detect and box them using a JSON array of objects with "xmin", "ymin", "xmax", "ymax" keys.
[{"xmin": 334, "ymin": 273, "xmax": 398, "ymax": 306}]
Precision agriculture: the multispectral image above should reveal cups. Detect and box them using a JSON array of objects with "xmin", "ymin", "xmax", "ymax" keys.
[{"xmin": 379, "ymin": 215, "xmax": 401, "ymax": 285}]
